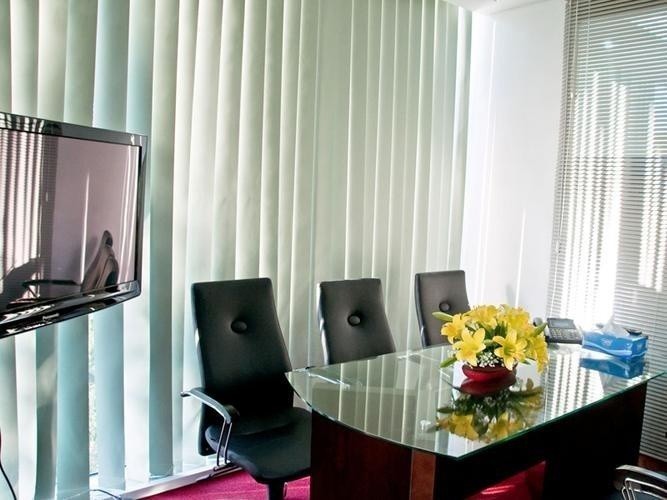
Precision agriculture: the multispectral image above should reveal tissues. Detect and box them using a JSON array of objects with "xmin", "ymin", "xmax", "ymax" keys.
[
  {"xmin": 579, "ymin": 314, "xmax": 649, "ymax": 358},
  {"xmin": 580, "ymin": 349, "xmax": 646, "ymax": 390}
]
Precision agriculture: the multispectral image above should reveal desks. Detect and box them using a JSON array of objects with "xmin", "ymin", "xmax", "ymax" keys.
[{"xmin": 283, "ymin": 339, "xmax": 666, "ymax": 500}]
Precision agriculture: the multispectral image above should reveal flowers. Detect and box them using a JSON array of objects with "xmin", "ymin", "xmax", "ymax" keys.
[
  {"xmin": 429, "ymin": 296, "xmax": 552, "ymax": 375},
  {"xmin": 428, "ymin": 379, "xmax": 545, "ymax": 444}
]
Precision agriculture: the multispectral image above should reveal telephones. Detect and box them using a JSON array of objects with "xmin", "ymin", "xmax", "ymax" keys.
[{"xmin": 534, "ymin": 317, "xmax": 583, "ymax": 344}]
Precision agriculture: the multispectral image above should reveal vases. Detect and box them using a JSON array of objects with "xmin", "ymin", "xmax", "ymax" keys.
[
  {"xmin": 461, "ymin": 379, "xmax": 516, "ymax": 395},
  {"xmin": 461, "ymin": 365, "xmax": 511, "ymax": 384}
]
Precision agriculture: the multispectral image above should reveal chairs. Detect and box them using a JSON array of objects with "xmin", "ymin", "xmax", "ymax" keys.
[
  {"xmin": 179, "ymin": 277, "xmax": 312, "ymax": 499},
  {"xmin": 414, "ymin": 270, "xmax": 472, "ymax": 348},
  {"xmin": 321, "ymin": 278, "xmax": 396, "ymax": 364}
]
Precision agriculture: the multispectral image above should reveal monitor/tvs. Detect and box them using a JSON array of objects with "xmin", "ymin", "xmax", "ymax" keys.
[{"xmin": 0, "ymin": 114, "xmax": 148, "ymax": 341}]
